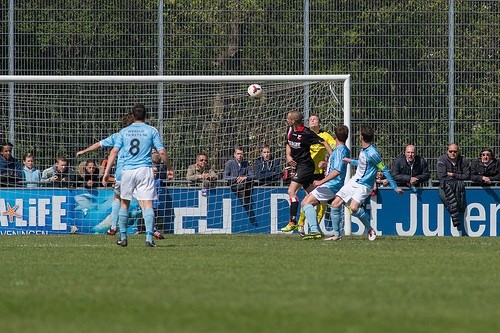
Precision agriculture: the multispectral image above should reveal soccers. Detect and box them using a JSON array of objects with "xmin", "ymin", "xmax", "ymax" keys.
[{"xmin": 247, "ymin": 84, "xmax": 263, "ymax": 97}]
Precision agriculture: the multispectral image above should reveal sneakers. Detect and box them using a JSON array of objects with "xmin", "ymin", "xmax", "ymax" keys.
[
  {"xmin": 146, "ymin": 240, "xmax": 158, "ymax": 247},
  {"xmin": 325, "ymin": 235, "xmax": 342, "ymax": 241},
  {"xmin": 302, "ymin": 231, "xmax": 322, "ymax": 240},
  {"xmin": 154, "ymin": 232, "xmax": 161, "ymax": 238},
  {"xmin": 298, "ymin": 221, "xmax": 304, "ymax": 233},
  {"xmin": 281, "ymin": 223, "xmax": 297, "ymax": 232},
  {"xmin": 368, "ymin": 228, "xmax": 376, "ymax": 241},
  {"xmin": 117, "ymin": 239, "xmax": 127, "ymax": 246},
  {"xmin": 107, "ymin": 226, "xmax": 116, "ymax": 235}
]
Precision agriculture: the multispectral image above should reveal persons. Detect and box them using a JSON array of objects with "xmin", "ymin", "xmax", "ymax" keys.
[
  {"xmin": 224, "ymin": 143, "xmax": 255, "ymax": 186},
  {"xmin": 391, "ymin": 145, "xmax": 431, "ymax": 187},
  {"xmin": 70, "ymin": 159, "xmax": 99, "ymax": 189},
  {"xmin": 186, "ymin": 153, "xmax": 217, "ymax": 187},
  {"xmin": 0, "ymin": 142, "xmax": 27, "ymax": 187},
  {"xmin": 281, "ymin": 110, "xmax": 333, "ymax": 232},
  {"xmin": 23, "ymin": 152, "xmax": 42, "ymax": 187},
  {"xmin": 471, "ymin": 147, "xmax": 500, "ymax": 186},
  {"xmin": 437, "ymin": 143, "xmax": 470, "ymax": 180},
  {"xmin": 324, "ymin": 125, "xmax": 405, "ymax": 239},
  {"xmin": 100, "ymin": 148, "xmax": 118, "ymax": 187},
  {"xmin": 255, "ymin": 146, "xmax": 281, "ymax": 186},
  {"xmin": 152, "ymin": 147, "xmax": 170, "ymax": 187},
  {"xmin": 76, "ymin": 104, "xmax": 174, "ymax": 248},
  {"xmin": 370, "ymin": 168, "xmax": 389, "ymax": 196},
  {"xmin": 41, "ymin": 156, "xmax": 68, "ymax": 187},
  {"xmin": 298, "ymin": 113, "xmax": 336, "ymax": 236},
  {"xmin": 301, "ymin": 124, "xmax": 350, "ymax": 240}
]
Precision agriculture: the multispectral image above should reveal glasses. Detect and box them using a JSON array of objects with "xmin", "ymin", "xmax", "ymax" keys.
[
  {"xmin": 448, "ymin": 149, "xmax": 457, "ymax": 153},
  {"xmin": 199, "ymin": 158, "xmax": 207, "ymax": 161},
  {"xmin": 482, "ymin": 153, "xmax": 490, "ymax": 156}
]
